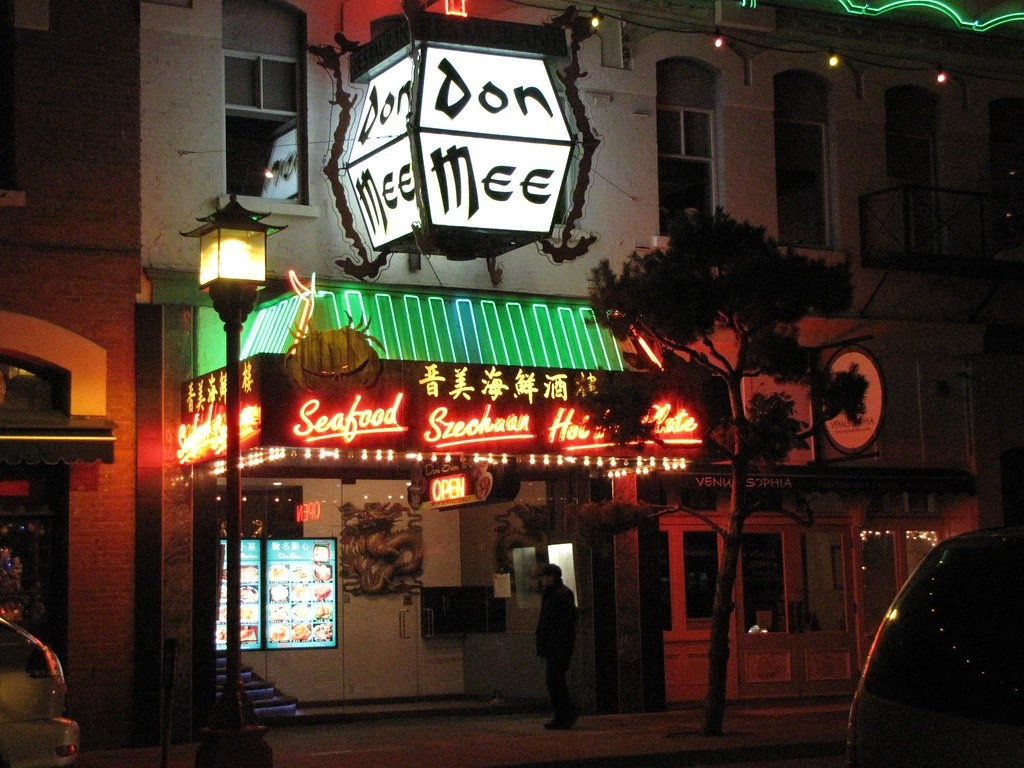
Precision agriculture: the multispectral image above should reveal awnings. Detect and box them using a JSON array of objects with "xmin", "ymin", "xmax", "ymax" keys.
[{"xmin": 0, "ymin": 409, "xmax": 118, "ymax": 464}]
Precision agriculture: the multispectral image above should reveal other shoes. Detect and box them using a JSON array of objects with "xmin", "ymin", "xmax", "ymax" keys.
[
  {"xmin": 544, "ymin": 718, "xmax": 571, "ymax": 730},
  {"xmin": 569, "ymin": 709, "xmax": 580, "ymax": 725}
]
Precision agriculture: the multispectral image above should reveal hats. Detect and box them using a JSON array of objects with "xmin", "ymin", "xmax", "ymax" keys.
[{"xmin": 537, "ymin": 564, "xmax": 561, "ymax": 576}]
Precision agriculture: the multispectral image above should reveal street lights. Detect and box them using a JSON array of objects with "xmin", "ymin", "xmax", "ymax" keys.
[{"xmin": 178, "ymin": 194, "xmax": 288, "ymax": 768}]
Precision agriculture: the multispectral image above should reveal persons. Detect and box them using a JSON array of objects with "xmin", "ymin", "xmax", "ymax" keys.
[{"xmin": 534, "ymin": 565, "xmax": 579, "ymax": 729}]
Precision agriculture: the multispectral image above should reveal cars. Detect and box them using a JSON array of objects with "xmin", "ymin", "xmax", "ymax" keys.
[
  {"xmin": 1, "ymin": 616, "xmax": 80, "ymax": 768},
  {"xmin": 843, "ymin": 526, "xmax": 1024, "ymax": 768}
]
